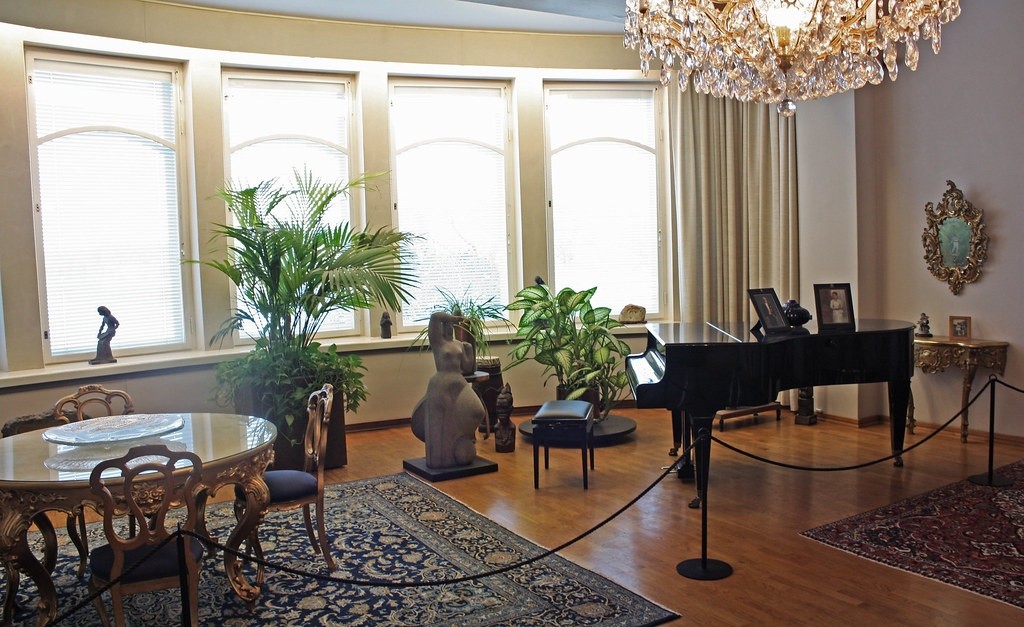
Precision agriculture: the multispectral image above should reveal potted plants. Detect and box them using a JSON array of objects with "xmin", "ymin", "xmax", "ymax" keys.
[
  {"xmin": 403, "ymin": 283, "xmax": 520, "ymax": 375},
  {"xmin": 178, "ymin": 160, "xmax": 427, "ymax": 474},
  {"xmin": 495, "ymin": 275, "xmax": 633, "ymax": 425}
]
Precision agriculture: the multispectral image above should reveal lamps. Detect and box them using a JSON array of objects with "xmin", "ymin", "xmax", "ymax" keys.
[{"xmin": 622, "ymin": 0, "xmax": 962, "ymax": 117}]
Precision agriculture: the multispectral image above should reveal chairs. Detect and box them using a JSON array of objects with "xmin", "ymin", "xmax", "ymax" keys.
[
  {"xmin": 233, "ymin": 383, "xmax": 337, "ymax": 588},
  {"xmin": 88, "ymin": 445, "xmax": 212, "ymax": 627},
  {"xmin": 55, "ymin": 384, "xmax": 158, "ymax": 577}
]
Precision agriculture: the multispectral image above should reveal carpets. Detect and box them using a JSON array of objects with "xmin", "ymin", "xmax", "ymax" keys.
[
  {"xmin": 0, "ymin": 470, "xmax": 682, "ymax": 627},
  {"xmin": 794, "ymin": 457, "xmax": 1024, "ymax": 612}
]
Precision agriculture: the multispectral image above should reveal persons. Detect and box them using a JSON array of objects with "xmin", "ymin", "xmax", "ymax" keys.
[
  {"xmin": 380, "ymin": 312, "xmax": 393, "ymax": 338},
  {"xmin": 495, "ymin": 384, "xmax": 516, "ymax": 452},
  {"xmin": 96, "ymin": 307, "xmax": 119, "ymax": 360},
  {"xmin": 956, "ymin": 322, "xmax": 966, "ymax": 335},
  {"xmin": 761, "ymin": 297, "xmax": 778, "ymax": 327},
  {"xmin": 831, "ymin": 293, "xmax": 844, "ymax": 322}
]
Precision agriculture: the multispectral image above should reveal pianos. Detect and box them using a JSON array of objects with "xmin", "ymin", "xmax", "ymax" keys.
[{"xmin": 624, "ymin": 317, "xmax": 915, "ymax": 509}]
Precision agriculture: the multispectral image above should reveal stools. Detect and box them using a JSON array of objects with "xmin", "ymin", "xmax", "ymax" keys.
[
  {"xmin": 462, "ymin": 371, "xmax": 490, "ymax": 441},
  {"xmin": 532, "ymin": 399, "xmax": 594, "ymax": 490}
]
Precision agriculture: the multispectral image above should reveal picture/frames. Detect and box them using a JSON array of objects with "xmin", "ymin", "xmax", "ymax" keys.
[
  {"xmin": 949, "ymin": 316, "xmax": 971, "ymax": 341},
  {"xmin": 813, "ymin": 283, "xmax": 856, "ymax": 332},
  {"xmin": 747, "ymin": 287, "xmax": 791, "ymax": 333},
  {"xmin": 920, "ymin": 179, "xmax": 991, "ymax": 297}
]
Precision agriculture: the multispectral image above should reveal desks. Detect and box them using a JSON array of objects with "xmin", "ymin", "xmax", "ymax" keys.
[
  {"xmin": 0, "ymin": 413, "xmax": 278, "ymax": 627},
  {"xmin": 906, "ymin": 335, "xmax": 1008, "ymax": 445}
]
